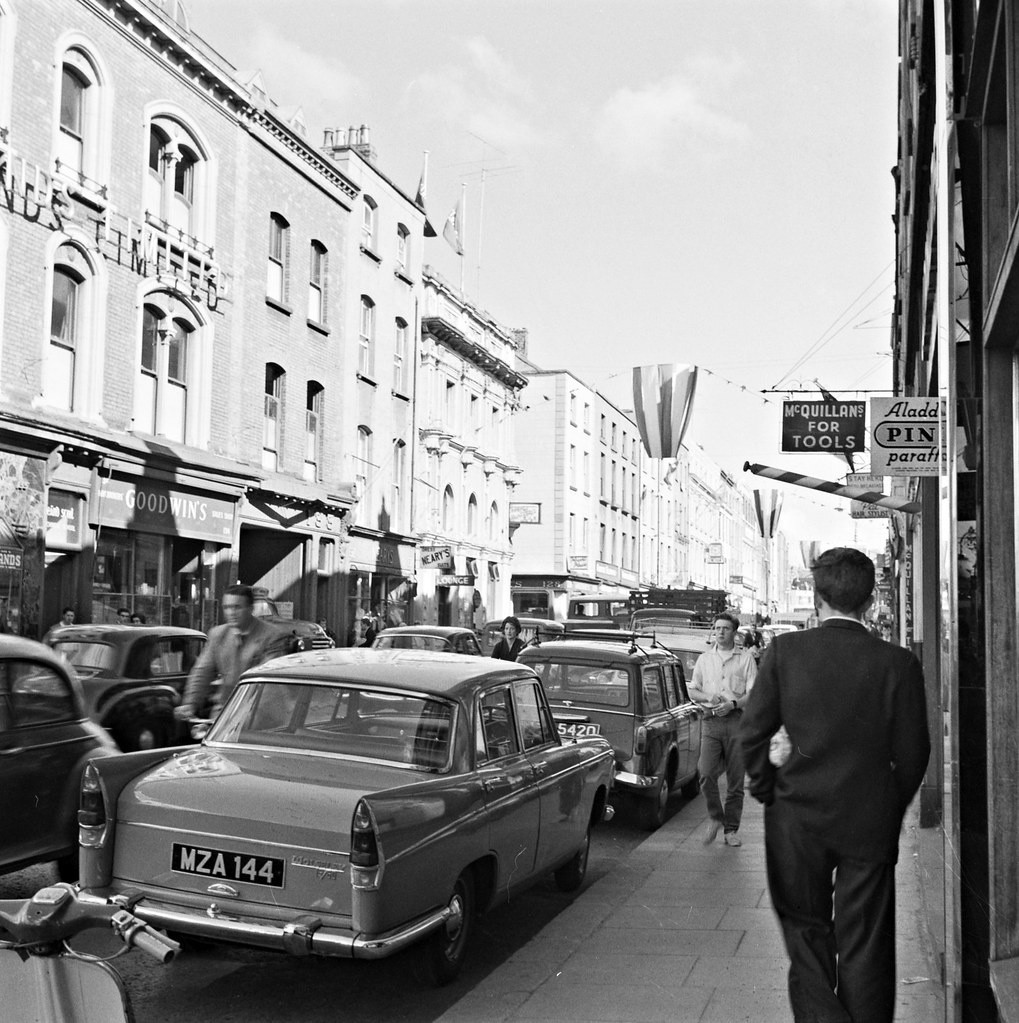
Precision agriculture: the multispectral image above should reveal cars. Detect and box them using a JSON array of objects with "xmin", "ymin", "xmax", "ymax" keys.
[
  {"xmin": 11, "ymin": 624, "xmax": 226, "ymax": 754},
  {"xmin": 0, "ymin": 633, "xmax": 125, "ymax": 901},
  {"xmin": 254, "ymin": 605, "xmax": 800, "ymax": 833},
  {"xmin": 107, "ymin": 645, "xmax": 615, "ymax": 987}
]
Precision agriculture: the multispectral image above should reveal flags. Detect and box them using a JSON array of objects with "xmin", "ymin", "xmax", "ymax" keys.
[
  {"xmin": 413, "ymin": 176, "xmax": 437, "ymax": 237},
  {"xmin": 442, "ymin": 197, "xmax": 464, "ymax": 256},
  {"xmin": 633, "ymin": 364, "xmax": 697, "ymax": 459}
]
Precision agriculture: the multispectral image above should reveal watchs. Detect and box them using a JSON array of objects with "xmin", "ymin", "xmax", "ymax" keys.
[{"xmin": 730, "ymin": 700, "xmax": 737, "ymax": 709}]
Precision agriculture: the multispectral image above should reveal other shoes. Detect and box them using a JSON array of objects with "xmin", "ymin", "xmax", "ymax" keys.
[
  {"xmin": 724, "ymin": 831, "xmax": 742, "ymax": 846},
  {"xmin": 705, "ymin": 819, "xmax": 722, "ymax": 843}
]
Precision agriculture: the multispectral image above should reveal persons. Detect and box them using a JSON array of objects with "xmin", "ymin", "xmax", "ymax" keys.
[
  {"xmin": 688, "ymin": 612, "xmax": 758, "ymax": 847},
  {"xmin": 742, "ymin": 624, "xmax": 764, "ymax": 651},
  {"xmin": 491, "ymin": 616, "xmax": 527, "ymax": 662},
  {"xmin": 131, "ymin": 613, "xmax": 147, "ymax": 625},
  {"xmin": 741, "ymin": 548, "xmax": 933, "ymax": 1023},
  {"xmin": 358, "ymin": 618, "xmax": 377, "ymax": 648},
  {"xmin": 319, "ymin": 618, "xmax": 330, "ymax": 637},
  {"xmin": 575, "ymin": 604, "xmax": 587, "ymax": 615},
  {"xmin": 116, "ymin": 607, "xmax": 130, "ymax": 624},
  {"xmin": 866, "ymin": 618, "xmax": 900, "ymax": 646},
  {"xmin": 174, "ymin": 585, "xmax": 290, "ymax": 732},
  {"xmin": 41, "ymin": 607, "xmax": 88, "ymax": 658}
]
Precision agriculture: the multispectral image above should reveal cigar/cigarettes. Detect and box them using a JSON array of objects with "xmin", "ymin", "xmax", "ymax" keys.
[{"xmin": 713, "ymin": 710, "xmax": 715, "ymax": 715}]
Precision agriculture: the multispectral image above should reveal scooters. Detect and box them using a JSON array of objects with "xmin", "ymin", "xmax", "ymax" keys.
[{"xmin": 0, "ymin": 880, "xmax": 185, "ymax": 1023}]
